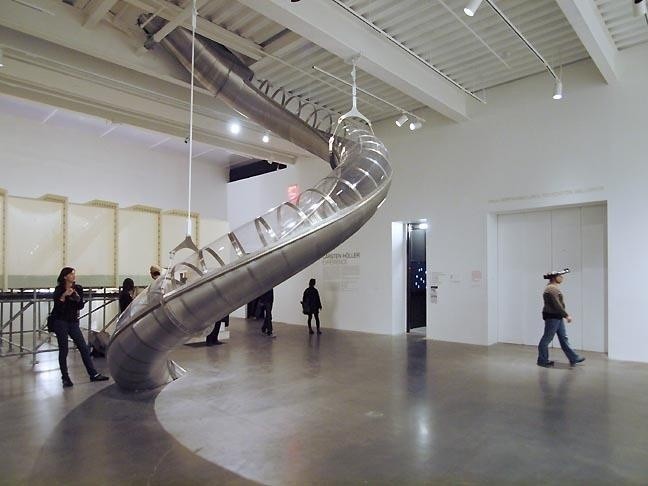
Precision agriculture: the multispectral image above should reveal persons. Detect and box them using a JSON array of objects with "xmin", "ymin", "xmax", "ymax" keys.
[
  {"xmin": 302, "ymin": 278, "xmax": 323, "ymax": 336},
  {"xmin": 119, "ymin": 277, "xmax": 135, "ymax": 312},
  {"xmin": 260, "ymin": 288, "xmax": 274, "ymax": 335},
  {"xmin": 48, "ymin": 267, "xmax": 109, "ymax": 386},
  {"xmin": 205, "ymin": 315, "xmax": 229, "ymax": 345},
  {"xmin": 537, "ymin": 268, "xmax": 585, "ymax": 367}
]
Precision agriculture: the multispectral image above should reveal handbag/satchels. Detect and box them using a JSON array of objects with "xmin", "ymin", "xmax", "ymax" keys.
[
  {"xmin": 47, "ymin": 316, "xmax": 55, "ymax": 332},
  {"xmin": 86, "ymin": 329, "xmax": 110, "ymax": 357}
]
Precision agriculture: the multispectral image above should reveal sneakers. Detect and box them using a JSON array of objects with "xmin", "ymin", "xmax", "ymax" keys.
[
  {"xmin": 576, "ymin": 358, "xmax": 585, "ymax": 363},
  {"xmin": 547, "ymin": 359, "xmax": 554, "ymax": 365},
  {"xmin": 63, "ymin": 378, "xmax": 73, "ymax": 386},
  {"xmin": 90, "ymin": 373, "xmax": 109, "ymax": 381}
]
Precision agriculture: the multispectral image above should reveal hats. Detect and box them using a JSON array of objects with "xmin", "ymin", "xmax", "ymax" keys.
[
  {"xmin": 150, "ymin": 264, "xmax": 160, "ymax": 272},
  {"xmin": 543, "ymin": 269, "xmax": 569, "ymax": 279}
]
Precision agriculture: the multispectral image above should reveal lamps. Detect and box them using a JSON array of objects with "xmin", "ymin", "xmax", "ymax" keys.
[
  {"xmin": 312, "ymin": 66, "xmax": 426, "ymax": 131},
  {"xmin": 463, "ymin": 1, "xmax": 563, "ymax": 100}
]
